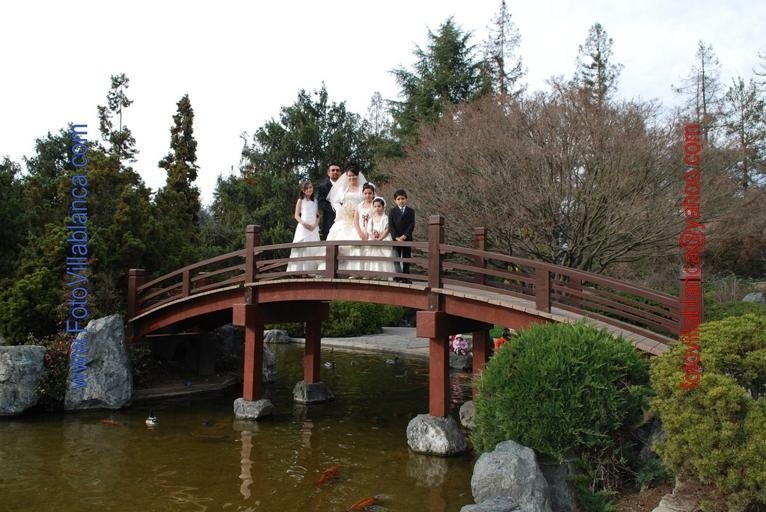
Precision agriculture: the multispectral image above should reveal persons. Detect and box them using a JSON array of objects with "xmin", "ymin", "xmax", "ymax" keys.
[
  {"xmin": 388, "ymin": 189, "xmax": 416, "ymax": 285},
  {"xmin": 449, "ymin": 328, "xmax": 511, "ymax": 358},
  {"xmin": 330, "ymin": 166, "xmax": 366, "ymax": 271},
  {"xmin": 315, "ymin": 163, "xmax": 343, "ymax": 241},
  {"xmin": 347, "ymin": 183, "xmax": 376, "ymax": 280},
  {"xmin": 366, "ymin": 197, "xmax": 392, "ymax": 281},
  {"xmin": 292, "ymin": 181, "xmax": 323, "ymax": 279}
]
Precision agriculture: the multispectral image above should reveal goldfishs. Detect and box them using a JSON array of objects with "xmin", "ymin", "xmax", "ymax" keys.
[
  {"xmin": 99, "ymin": 420, "xmax": 122, "ymax": 427},
  {"xmin": 196, "ymin": 433, "xmax": 229, "ymax": 440},
  {"xmin": 311, "ymin": 464, "xmax": 342, "ymax": 487},
  {"xmin": 346, "ymin": 496, "xmax": 378, "ymax": 512}
]
